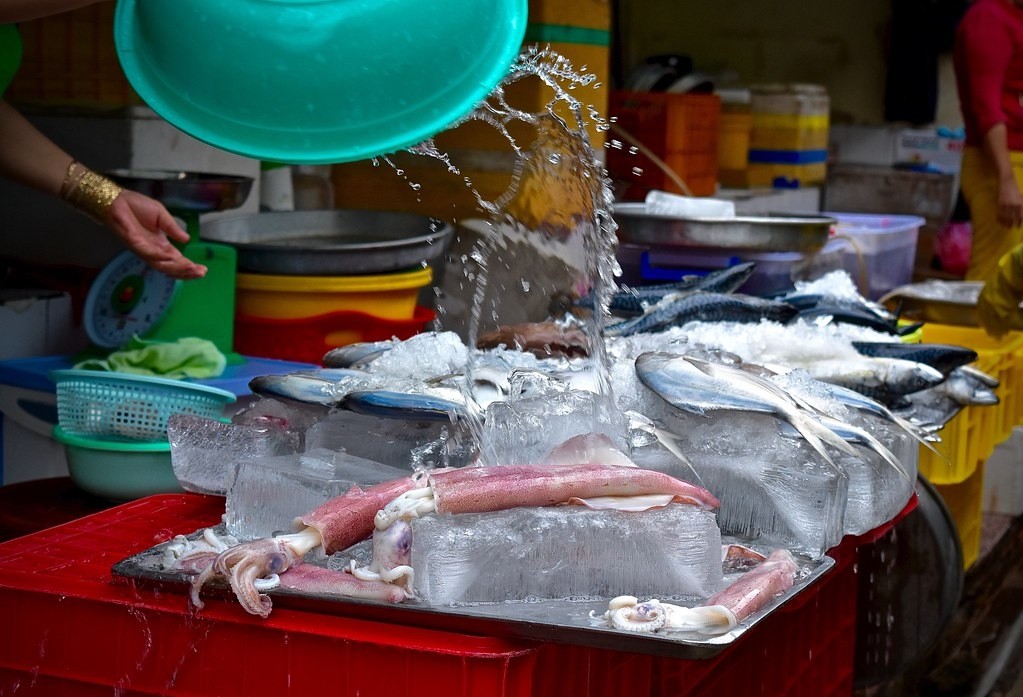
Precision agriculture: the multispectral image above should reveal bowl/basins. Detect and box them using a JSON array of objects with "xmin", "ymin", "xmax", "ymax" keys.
[
  {"xmin": 51, "ymin": 418, "xmax": 233, "ymax": 502},
  {"xmin": 235, "ymin": 268, "xmax": 433, "ymax": 341},
  {"xmin": 115, "ymin": 0, "xmax": 531, "ymax": 166}
]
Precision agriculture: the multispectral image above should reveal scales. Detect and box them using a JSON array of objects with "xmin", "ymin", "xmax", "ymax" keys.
[{"xmin": 85, "ymin": 169, "xmax": 255, "ymax": 367}]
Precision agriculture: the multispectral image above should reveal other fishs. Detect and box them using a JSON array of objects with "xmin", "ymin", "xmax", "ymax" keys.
[{"xmin": 247, "ymin": 260, "xmax": 1003, "ymax": 479}]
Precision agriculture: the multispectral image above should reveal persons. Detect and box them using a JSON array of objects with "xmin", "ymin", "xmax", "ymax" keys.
[
  {"xmin": 0, "ymin": 99, "xmax": 208, "ymax": 279},
  {"xmin": 953, "ymin": 0, "xmax": 1023, "ymax": 281}
]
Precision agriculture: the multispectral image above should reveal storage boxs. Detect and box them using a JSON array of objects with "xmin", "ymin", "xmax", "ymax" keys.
[{"xmin": 0, "ymin": 94, "xmax": 1023, "ymax": 697}]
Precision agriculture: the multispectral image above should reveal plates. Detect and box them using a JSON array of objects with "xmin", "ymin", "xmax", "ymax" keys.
[{"xmin": 198, "ymin": 207, "xmax": 450, "ymax": 274}]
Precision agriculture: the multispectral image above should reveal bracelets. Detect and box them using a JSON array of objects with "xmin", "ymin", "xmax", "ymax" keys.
[{"xmin": 59, "ymin": 158, "xmax": 122, "ymax": 225}]
